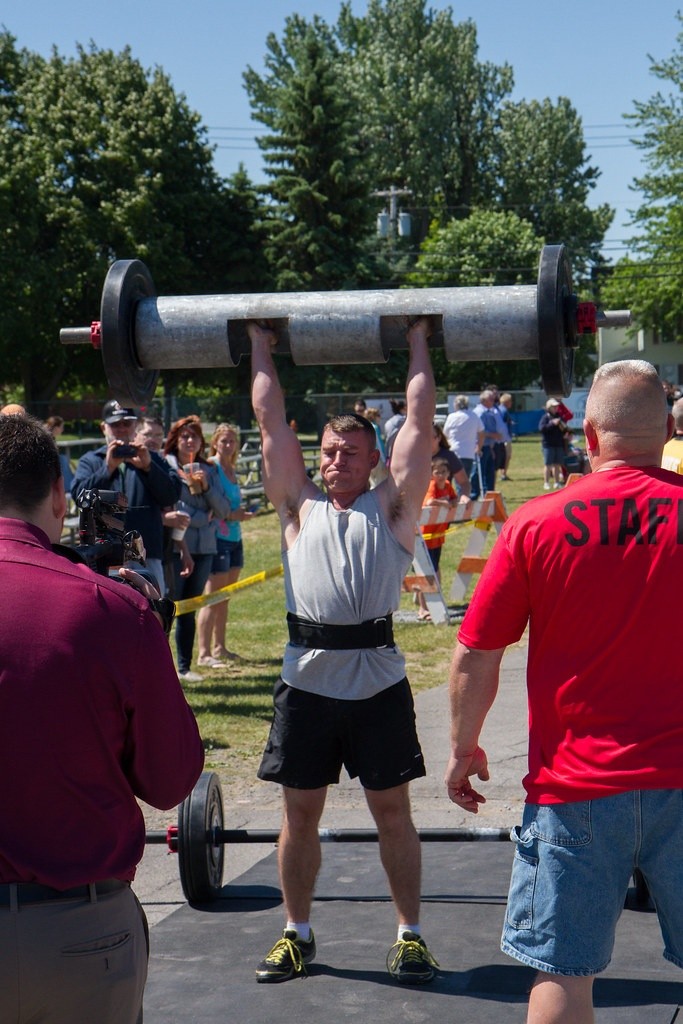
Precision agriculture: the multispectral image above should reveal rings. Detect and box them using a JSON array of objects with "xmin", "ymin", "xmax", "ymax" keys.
[{"xmin": 462, "ymin": 793, "xmax": 465, "ymax": 796}]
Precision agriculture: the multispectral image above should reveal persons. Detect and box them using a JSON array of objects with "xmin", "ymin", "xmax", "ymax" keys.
[
  {"xmin": 0, "ymin": 401, "xmax": 206, "ymax": 1024},
  {"xmin": 446, "ymin": 359, "xmax": 683, "ymax": 1024},
  {"xmin": 248, "ymin": 313, "xmax": 438, "ymax": 982},
  {"xmin": 69, "ymin": 395, "xmax": 261, "ymax": 682},
  {"xmin": 350, "ymin": 395, "xmax": 472, "ymax": 622}
]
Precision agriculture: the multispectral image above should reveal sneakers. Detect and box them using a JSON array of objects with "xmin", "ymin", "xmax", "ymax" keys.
[
  {"xmin": 386, "ymin": 931, "xmax": 440, "ymax": 985},
  {"xmin": 255, "ymin": 926, "xmax": 316, "ymax": 984}
]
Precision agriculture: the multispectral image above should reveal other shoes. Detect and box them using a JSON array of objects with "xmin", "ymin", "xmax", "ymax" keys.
[
  {"xmin": 553, "ymin": 483, "xmax": 558, "ymax": 489},
  {"xmin": 197, "ymin": 657, "xmax": 231, "ymax": 669},
  {"xmin": 213, "ymin": 650, "xmax": 243, "ymax": 661},
  {"xmin": 499, "ymin": 474, "xmax": 512, "ymax": 481},
  {"xmin": 543, "ymin": 484, "xmax": 550, "ymax": 490},
  {"xmin": 410, "ymin": 584, "xmax": 421, "ymax": 606},
  {"xmin": 176, "ymin": 670, "xmax": 204, "ymax": 683},
  {"xmin": 417, "ymin": 609, "xmax": 432, "ymax": 621}
]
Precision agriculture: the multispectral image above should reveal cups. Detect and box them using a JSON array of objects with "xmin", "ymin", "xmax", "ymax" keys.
[
  {"xmin": 172, "ymin": 511, "xmax": 190, "ymax": 543},
  {"xmin": 183, "ymin": 462, "xmax": 202, "ymax": 495}
]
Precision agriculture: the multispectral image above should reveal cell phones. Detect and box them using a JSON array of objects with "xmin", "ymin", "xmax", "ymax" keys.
[{"xmin": 112, "ymin": 446, "xmax": 138, "ymax": 459}]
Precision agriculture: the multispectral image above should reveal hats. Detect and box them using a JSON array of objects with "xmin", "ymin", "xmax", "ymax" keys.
[
  {"xmin": 102, "ymin": 399, "xmax": 137, "ymax": 423},
  {"xmin": 546, "ymin": 398, "xmax": 559, "ymax": 411}
]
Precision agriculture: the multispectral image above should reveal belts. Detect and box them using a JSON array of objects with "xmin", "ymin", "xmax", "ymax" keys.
[{"xmin": 0, "ymin": 876, "xmax": 131, "ymax": 907}]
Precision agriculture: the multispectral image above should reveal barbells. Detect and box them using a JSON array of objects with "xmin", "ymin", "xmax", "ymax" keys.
[
  {"xmin": 145, "ymin": 770, "xmax": 655, "ymax": 911},
  {"xmin": 58, "ymin": 242, "xmax": 638, "ymax": 402}
]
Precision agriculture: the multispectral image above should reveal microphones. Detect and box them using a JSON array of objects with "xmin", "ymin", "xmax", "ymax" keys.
[{"xmin": 127, "ymin": 530, "xmax": 146, "ymax": 562}]
